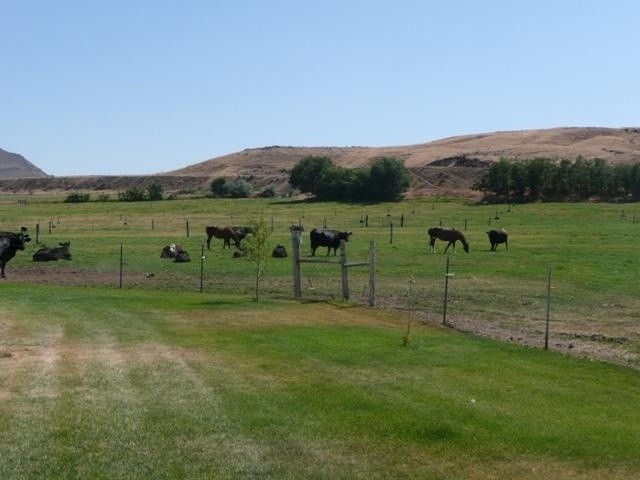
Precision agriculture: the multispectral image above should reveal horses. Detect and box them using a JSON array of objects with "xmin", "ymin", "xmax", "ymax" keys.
[
  {"xmin": 428, "ymin": 227, "xmax": 470, "ymax": 254},
  {"xmin": 226, "ymin": 225, "xmax": 256, "ymax": 245},
  {"xmin": 206, "ymin": 224, "xmax": 242, "ymax": 251}
]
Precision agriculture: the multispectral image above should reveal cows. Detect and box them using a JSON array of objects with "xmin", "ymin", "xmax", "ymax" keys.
[
  {"xmin": 486, "ymin": 228, "xmax": 508, "ymax": 250},
  {"xmin": 33, "ymin": 240, "xmax": 72, "ymax": 262},
  {"xmin": 0, "ymin": 226, "xmax": 32, "ymax": 278},
  {"xmin": 272, "ymin": 244, "xmax": 287, "ymax": 257},
  {"xmin": 233, "ymin": 248, "xmax": 247, "ymax": 257},
  {"xmin": 310, "ymin": 228, "xmax": 352, "ymax": 256},
  {"xmin": 160, "ymin": 243, "xmax": 191, "ymax": 263}
]
